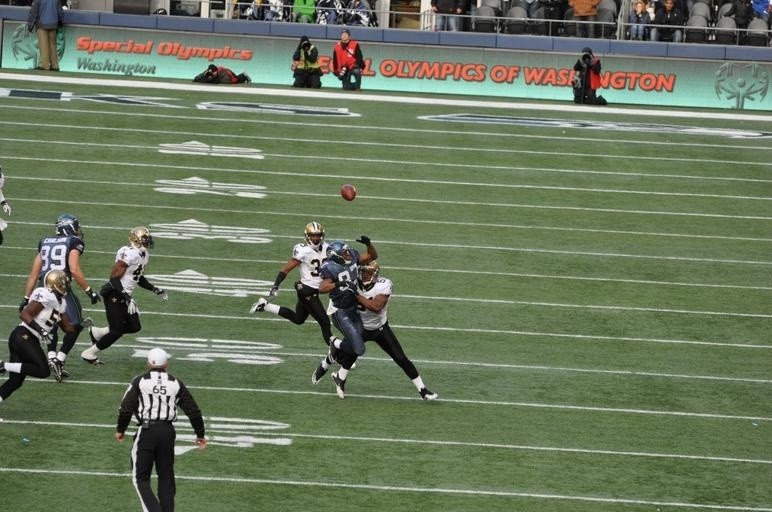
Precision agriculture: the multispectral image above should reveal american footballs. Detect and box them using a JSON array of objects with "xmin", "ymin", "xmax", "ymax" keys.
[{"xmin": 340, "ymin": 183, "xmax": 357, "ymax": 201}]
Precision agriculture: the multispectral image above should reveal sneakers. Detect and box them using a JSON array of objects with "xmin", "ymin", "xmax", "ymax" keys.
[
  {"xmin": 249, "ymin": 297, "xmax": 267, "ymax": 313},
  {"xmin": 47, "ymin": 357, "xmax": 62, "ymax": 382},
  {"xmin": 80, "ymin": 351, "xmax": 104, "ymax": 366},
  {"xmin": 328, "ymin": 336, "xmax": 339, "ymax": 366},
  {"xmin": 60, "ymin": 360, "xmax": 69, "ymax": 377},
  {"xmin": 312, "ymin": 364, "xmax": 328, "ymax": 385},
  {"xmin": 419, "ymin": 387, "xmax": 437, "ymax": 401},
  {"xmin": 331, "ymin": 372, "xmax": 346, "ymax": 400},
  {"xmin": 88, "ymin": 326, "xmax": 98, "ymax": 346}
]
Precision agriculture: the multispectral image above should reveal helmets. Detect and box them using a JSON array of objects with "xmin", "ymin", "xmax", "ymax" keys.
[
  {"xmin": 129, "ymin": 226, "xmax": 154, "ymax": 249},
  {"xmin": 55, "ymin": 214, "xmax": 80, "ymax": 237},
  {"xmin": 358, "ymin": 260, "xmax": 380, "ymax": 292},
  {"xmin": 43, "ymin": 269, "xmax": 71, "ymax": 297},
  {"xmin": 304, "ymin": 221, "xmax": 325, "ymax": 249},
  {"xmin": 326, "ymin": 240, "xmax": 353, "ymax": 266}
]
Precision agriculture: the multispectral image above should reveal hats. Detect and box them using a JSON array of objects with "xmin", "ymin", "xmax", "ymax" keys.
[{"xmin": 148, "ymin": 349, "xmax": 167, "ymax": 366}]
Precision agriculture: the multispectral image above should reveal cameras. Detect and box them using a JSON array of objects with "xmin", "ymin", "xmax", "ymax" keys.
[
  {"xmin": 301, "ymin": 41, "xmax": 311, "ymax": 50},
  {"xmin": 580, "ymin": 52, "xmax": 593, "ymax": 64},
  {"xmin": 205, "ymin": 72, "xmax": 213, "ymax": 81}
]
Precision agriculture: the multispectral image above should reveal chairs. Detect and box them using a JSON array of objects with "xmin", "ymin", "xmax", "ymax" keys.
[
  {"xmin": 471, "ymin": 0, "xmax": 617, "ymax": 39},
  {"xmin": 683, "ymin": 0, "xmax": 769, "ymax": 46}
]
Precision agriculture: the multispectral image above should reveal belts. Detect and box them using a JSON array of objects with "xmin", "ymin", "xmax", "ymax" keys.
[{"xmin": 149, "ymin": 421, "xmax": 166, "ymax": 425}]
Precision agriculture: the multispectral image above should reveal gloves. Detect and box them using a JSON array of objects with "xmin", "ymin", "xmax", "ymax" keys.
[
  {"xmin": 40, "ymin": 329, "xmax": 53, "ymax": 344},
  {"xmin": 19, "ymin": 298, "xmax": 28, "ymax": 313},
  {"xmin": 356, "ymin": 236, "xmax": 370, "ymax": 246},
  {"xmin": 80, "ymin": 317, "xmax": 94, "ymax": 327},
  {"xmin": 339, "ymin": 283, "xmax": 357, "ymax": 295},
  {"xmin": 2, "ymin": 203, "xmax": 11, "ymax": 216},
  {"xmin": 266, "ymin": 288, "xmax": 278, "ymax": 301},
  {"xmin": 86, "ymin": 288, "xmax": 101, "ymax": 304},
  {"xmin": 127, "ymin": 298, "xmax": 136, "ymax": 315},
  {"xmin": 152, "ymin": 287, "xmax": 165, "ymax": 297}
]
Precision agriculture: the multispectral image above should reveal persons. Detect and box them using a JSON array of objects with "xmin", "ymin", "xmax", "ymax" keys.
[
  {"xmin": 21, "ymin": 213, "xmax": 101, "ymax": 375},
  {"xmin": 316, "ymin": 235, "xmax": 379, "ymax": 400},
  {"xmin": 116, "ymin": 349, "xmax": 207, "ymax": 512},
  {"xmin": 194, "ymin": 64, "xmax": 251, "ymax": 83},
  {"xmin": 0, "ymin": 166, "xmax": 14, "ymax": 244},
  {"xmin": 2, "ymin": 269, "xmax": 94, "ymax": 403},
  {"xmin": 248, "ymin": 222, "xmax": 333, "ymax": 346},
  {"xmin": 291, "ymin": 37, "xmax": 323, "ymax": 89},
  {"xmin": 331, "ymin": 29, "xmax": 365, "ymax": 89},
  {"xmin": 310, "ymin": 261, "xmax": 439, "ymax": 402},
  {"xmin": 570, "ymin": 47, "xmax": 606, "ymax": 106},
  {"xmin": 26, "ymin": 0, "xmax": 65, "ymax": 70},
  {"xmin": 81, "ymin": 225, "xmax": 167, "ymax": 366}
]
